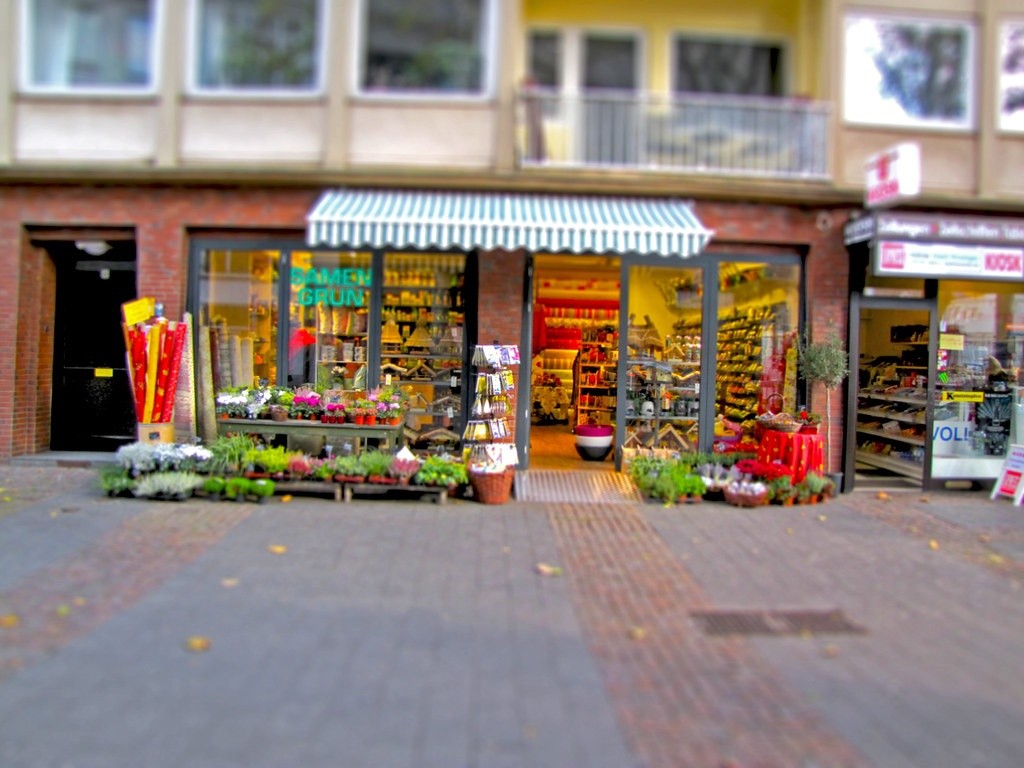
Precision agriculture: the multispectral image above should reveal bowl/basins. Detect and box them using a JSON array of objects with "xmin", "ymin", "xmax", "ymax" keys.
[{"xmin": 575, "ymin": 424, "xmax": 614, "ymax": 461}]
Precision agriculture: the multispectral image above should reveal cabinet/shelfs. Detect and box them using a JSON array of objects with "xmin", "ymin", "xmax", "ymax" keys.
[
  {"xmin": 668, "ymin": 299, "xmax": 788, "ymax": 423},
  {"xmin": 854, "ymin": 337, "xmax": 970, "ymax": 493},
  {"xmin": 619, "ymin": 357, "xmax": 701, "ymax": 451},
  {"xmin": 215, "ymin": 250, "xmax": 300, "ymax": 384},
  {"xmin": 378, "ymin": 353, "xmax": 462, "ymax": 417},
  {"xmin": 318, "ymin": 282, "xmax": 454, "ymax": 339},
  {"xmin": 571, "ymin": 338, "xmax": 617, "ymax": 426},
  {"xmin": 317, "ymin": 331, "xmax": 368, "ymax": 394}
]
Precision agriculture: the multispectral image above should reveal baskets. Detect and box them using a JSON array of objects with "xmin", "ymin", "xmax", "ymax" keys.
[{"xmin": 469, "ymin": 464, "xmax": 515, "ymax": 505}]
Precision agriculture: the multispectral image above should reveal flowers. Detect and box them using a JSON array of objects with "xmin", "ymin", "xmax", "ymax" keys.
[
  {"xmin": 795, "ymin": 410, "xmax": 822, "ymax": 424},
  {"xmin": 288, "ymin": 454, "xmax": 336, "ymax": 478},
  {"xmin": 212, "ymin": 381, "xmax": 412, "ymax": 419},
  {"xmin": 329, "ymin": 366, "xmax": 349, "ymax": 382},
  {"xmin": 114, "ymin": 439, "xmax": 214, "ymax": 476}
]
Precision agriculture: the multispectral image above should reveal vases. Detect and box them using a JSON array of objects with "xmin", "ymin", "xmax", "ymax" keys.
[
  {"xmin": 801, "ymin": 425, "xmax": 820, "ymax": 434},
  {"xmin": 235, "ymin": 413, "xmax": 243, "ymax": 418},
  {"xmin": 219, "ymin": 414, "xmax": 229, "ymax": 420},
  {"xmin": 389, "ymin": 417, "xmax": 402, "ymax": 425},
  {"xmin": 367, "ymin": 416, "xmax": 376, "ymax": 425},
  {"xmin": 354, "ymin": 416, "xmax": 364, "ymax": 424},
  {"xmin": 331, "ymin": 383, "xmax": 344, "ymax": 390},
  {"xmin": 328, "ymin": 416, "xmax": 336, "ymax": 423},
  {"xmin": 310, "ymin": 414, "xmax": 317, "ymax": 420},
  {"xmin": 296, "ymin": 416, "xmax": 302, "ymax": 420},
  {"xmin": 272, "ymin": 411, "xmax": 288, "ymax": 422},
  {"xmin": 260, "ymin": 413, "xmax": 268, "ymax": 419},
  {"xmin": 321, "ymin": 415, "xmax": 328, "ymax": 423},
  {"xmin": 379, "ymin": 418, "xmax": 387, "ymax": 425},
  {"xmin": 336, "ymin": 416, "xmax": 344, "ymax": 424},
  {"xmin": 323, "ymin": 475, "xmax": 333, "ymax": 484},
  {"xmin": 347, "ymin": 414, "xmax": 355, "ymax": 423},
  {"xmin": 316, "ymin": 478, "xmax": 324, "ymax": 482}
]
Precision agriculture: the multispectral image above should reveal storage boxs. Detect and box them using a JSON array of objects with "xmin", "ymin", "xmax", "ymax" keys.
[
  {"xmin": 654, "ymin": 449, "xmax": 668, "ymax": 459},
  {"xmin": 668, "ymin": 449, "xmax": 679, "ymax": 465},
  {"xmin": 640, "ymin": 449, "xmax": 652, "ymax": 456},
  {"xmin": 621, "ymin": 448, "xmax": 636, "ymax": 475}
]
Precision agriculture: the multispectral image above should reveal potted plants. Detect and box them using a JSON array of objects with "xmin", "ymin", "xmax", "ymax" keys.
[
  {"xmin": 203, "ymin": 477, "xmax": 225, "ymax": 501},
  {"xmin": 772, "ymin": 328, "xmax": 852, "ymax": 512},
  {"xmin": 226, "ymin": 477, "xmax": 250, "ymax": 503},
  {"xmin": 684, "ymin": 452, "xmax": 739, "ymax": 469},
  {"xmin": 250, "ymin": 478, "xmax": 276, "ymax": 506},
  {"xmin": 206, "ymin": 430, "xmax": 302, "ymax": 479},
  {"xmin": 134, "ymin": 469, "xmax": 204, "ymax": 501},
  {"xmin": 335, "ymin": 449, "xmax": 470, "ymax": 491},
  {"xmin": 96, "ymin": 464, "xmax": 137, "ymax": 498},
  {"xmin": 642, "ymin": 474, "xmax": 708, "ymax": 509}
]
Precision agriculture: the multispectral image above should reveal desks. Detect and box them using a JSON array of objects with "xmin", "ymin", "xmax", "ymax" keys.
[
  {"xmin": 763, "ymin": 431, "xmax": 824, "ymax": 482},
  {"xmin": 217, "ymin": 418, "xmax": 408, "ymax": 452}
]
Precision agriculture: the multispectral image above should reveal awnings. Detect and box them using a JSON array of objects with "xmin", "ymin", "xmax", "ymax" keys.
[{"xmin": 305, "ymin": 188, "xmax": 716, "ymax": 260}]
